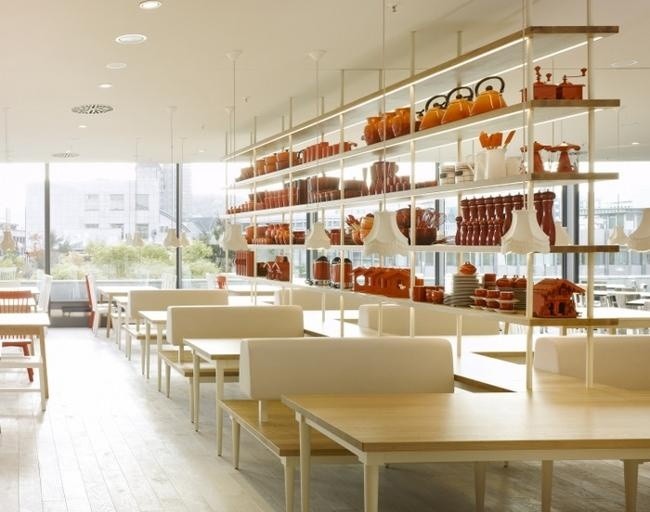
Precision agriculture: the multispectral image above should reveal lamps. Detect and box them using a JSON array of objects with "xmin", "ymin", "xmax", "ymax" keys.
[
  {"xmin": 363, "ymin": 1, "xmax": 412, "ymax": 258},
  {"xmin": 500, "ymin": 1, "xmax": 552, "ymax": 254},
  {"xmin": 106, "ymin": 61, "xmax": 126, "ymax": 69},
  {"xmin": 116, "ymin": 33, "xmax": 146, "ymax": 45},
  {"xmin": 221, "ymin": 51, "xmax": 248, "ymax": 251},
  {"xmin": 554, "ymin": 221, "xmax": 570, "ymax": 247},
  {"xmin": 627, "ymin": 207, "xmax": 650, "ymax": 252},
  {"xmin": 164, "ymin": 106, "xmax": 179, "ymax": 247},
  {"xmin": 96, "ymin": 82, "xmax": 111, "ymax": 88},
  {"xmin": 139, "ymin": 1, "xmax": 162, "ymax": 9},
  {"xmin": 607, "ymin": 107, "xmax": 632, "ymax": 248},
  {"xmin": 305, "ymin": 50, "xmax": 331, "ymax": 249},
  {"xmin": 610, "ymin": 58, "xmax": 638, "ymax": 67}
]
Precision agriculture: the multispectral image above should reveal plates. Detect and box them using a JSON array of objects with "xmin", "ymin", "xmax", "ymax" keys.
[{"xmin": 444, "ymin": 275, "xmax": 481, "ymax": 310}]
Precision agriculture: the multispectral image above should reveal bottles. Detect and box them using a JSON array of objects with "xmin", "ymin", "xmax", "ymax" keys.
[
  {"xmin": 363, "ymin": 108, "xmax": 410, "ymax": 146},
  {"xmin": 235, "ymin": 250, "xmax": 253, "ymax": 278},
  {"xmin": 264, "ymin": 222, "xmax": 289, "ymax": 245},
  {"xmin": 454, "ymin": 190, "xmax": 558, "ymax": 247},
  {"xmin": 437, "ymin": 147, "xmax": 526, "ymax": 187},
  {"xmin": 360, "ymin": 160, "xmax": 411, "ymax": 197}
]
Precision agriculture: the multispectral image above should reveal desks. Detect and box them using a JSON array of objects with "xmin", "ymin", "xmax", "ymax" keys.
[{"xmin": 0, "ymin": 313, "xmax": 51, "ymax": 411}]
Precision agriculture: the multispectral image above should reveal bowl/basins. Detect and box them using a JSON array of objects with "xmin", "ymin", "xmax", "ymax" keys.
[
  {"xmin": 238, "ymin": 148, "xmax": 300, "ymax": 181},
  {"xmin": 409, "ymin": 225, "xmax": 438, "ymax": 246},
  {"xmin": 247, "ymin": 191, "xmax": 265, "ymax": 211}
]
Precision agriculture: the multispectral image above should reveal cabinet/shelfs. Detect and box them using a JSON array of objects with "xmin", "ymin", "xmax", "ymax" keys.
[{"xmin": 220, "ymin": 0, "xmax": 620, "ymax": 390}]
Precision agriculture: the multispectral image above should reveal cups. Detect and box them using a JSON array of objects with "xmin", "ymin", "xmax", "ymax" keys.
[
  {"xmin": 263, "ymin": 187, "xmax": 297, "ymax": 211},
  {"xmin": 482, "ymin": 273, "xmax": 497, "ymax": 291},
  {"xmin": 413, "ymin": 285, "xmax": 444, "ymax": 302},
  {"xmin": 226, "ymin": 201, "xmax": 253, "ymax": 214},
  {"xmin": 330, "ymin": 229, "xmax": 342, "ymax": 246},
  {"xmin": 216, "ymin": 276, "xmax": 227, "ymax": 290},
  {"xmin": 468, "ymin": 288, "xmax": 520, "ymax": 314},
  {"xmin": 283, "ymin": 176, "xmax": 361, "ymax": 204},
  {"xmin": 293, "ymin": 231, "xmax": 305, "ymax": 244},
  {"xmin": 301, "ymin": 141, "xmax": 350, "ymax": 164}
]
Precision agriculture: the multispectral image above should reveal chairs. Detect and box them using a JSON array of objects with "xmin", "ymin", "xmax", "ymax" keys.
[
  {"xmin": 85, "ymin": 274, "xmax": 117, "ymax": 337},
  {"xmin": 573, "ymin": 279, "xmax": 650, "ymax": 334},
  {"xmin": 0, "ymin": 269, "xmax": 54, "ymax": 382}
]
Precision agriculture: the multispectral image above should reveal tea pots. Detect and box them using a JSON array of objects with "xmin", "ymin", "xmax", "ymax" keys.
[
  {"xmin": 495, "ymin": 273, "xmax": 528, "ymax": 288},
  {"xmin": 312, "ymin": 256, "xmax": 353, "ymax": 290},
  {"xmin": 416, "ymin": 75, "xmax": 508, "ymax": 132},
  {"xmin": 458, "ymin": 260, "xmax": 477, "ymax": 275}
]
